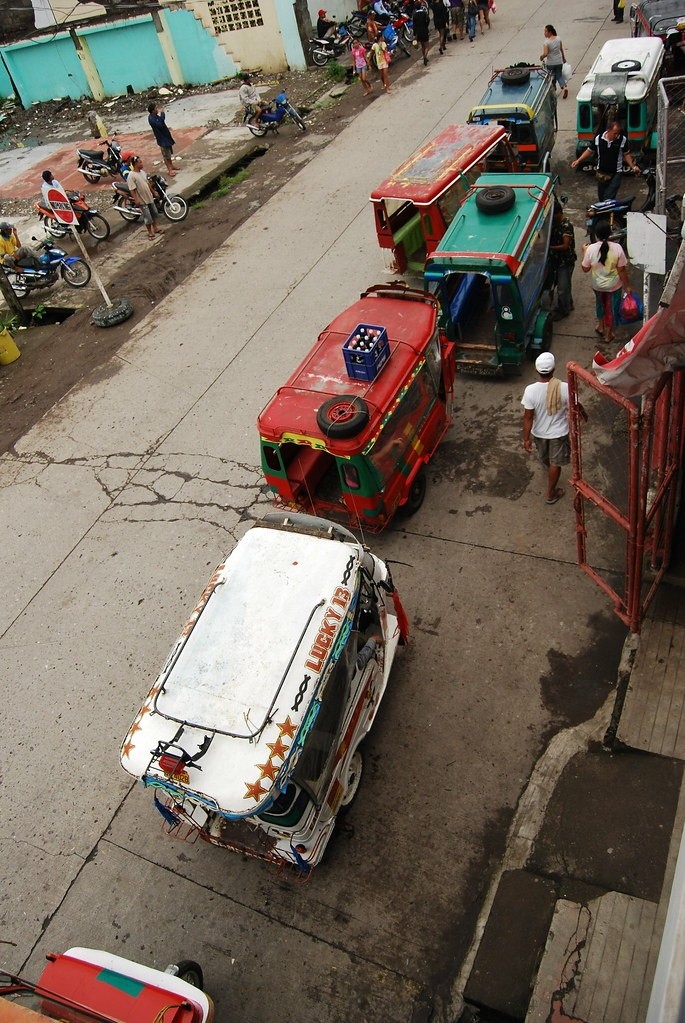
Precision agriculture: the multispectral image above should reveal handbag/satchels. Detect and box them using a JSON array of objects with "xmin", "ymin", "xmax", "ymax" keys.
[
  {"xmin": 468, "ymin": 0, "xmax": 496, "ymax": 16},
  {"xmin": 383, "ymin": 50, "xmax": 391, "ymax": 64},
  {"xmin": 562, "ymin": 62, "xmax": 572, "ymax": 81},
  {"xmin": 618, "ymin": 0, "xmax": 625, "ymax": 8},
  {"xmin": 619, "ymin": 289, "xmax": 644, "ymax": 325},
  {"xmin": 413, "ymin": 39, "xmax": 420, "ymax": 50},
  {"xmin": 595, "ymin": 171, "xmax": 620, "ymax": 184}
]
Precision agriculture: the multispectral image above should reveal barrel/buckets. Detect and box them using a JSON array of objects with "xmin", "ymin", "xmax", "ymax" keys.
[{"xmin": 0, "ymin": 326, "xmax": 21, "ymax": 366}]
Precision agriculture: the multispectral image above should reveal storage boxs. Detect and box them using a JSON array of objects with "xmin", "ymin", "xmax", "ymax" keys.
[
  {"xmin": 342, "ymin": 323, "xmax": 389, "ymax": 364},
  {"xmin": 345, "ymin": 344, "xmax": 391, "ymax": 381}
]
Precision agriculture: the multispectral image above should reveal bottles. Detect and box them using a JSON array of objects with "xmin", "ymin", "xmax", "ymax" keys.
[{"xmin": 347, "ymin": 328, "xmax": 383, "ymax": 364}]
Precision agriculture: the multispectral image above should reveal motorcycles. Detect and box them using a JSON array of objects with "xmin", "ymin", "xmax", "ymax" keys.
[
  {"xmin": 243, "ymin": 87, "xmax": 307, "ymax": 138},
  {"xmin": 307, "ymin": 15, "xmax": 364, "ymax": 67},
  {"xmin": 76, "ymin": 129, "xmax": 124, "ymax": 185},
  {"xmin": 34, "ymin": 189, "xmax": 111, "ymax": 241},
  {"xmin": 369, "ymin": 125, "xmax": 520, "ymax": 279},
  {"xmin": 1, "ymin": 228, "xmax": 91, "ymax": 301},
  {"xmin": 118, "ymin": 511, "xmax": 412, "ymax": 884},
  {"xmin": 584, "ymin": 157, "xmax": 684, "ymax": 249},
  {"xmin": 0, "ymin": 940, "xmax": 216, "ymax": 1023},
  {"xmin": 111, "ymin": 173, "xmax": 189, "ymax": 223},
  {"xmin": 346, "ymin": 0, "xmax": 429, "ymax": 71},
  {"xmin": 257, "ymin": 282, "xmax": 457, "ymax": 535},
  {"xmin": 466, "ymin": 60, "xmax": 560, "ymax": 173},
  {"xmin": 575, "ymin": 35, "xmax": 666, "ymax": 170},
  {"xmin": 423, "ymin": 172, "xmax": 569, "ymax": 377},
  {"xmin": 629, "ymin": 0, "xmax": 685, "ymax": 109}
]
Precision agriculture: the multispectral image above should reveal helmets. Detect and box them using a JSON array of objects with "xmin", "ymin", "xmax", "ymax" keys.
[{"xmin": 367, "ymin": 10, "xmax": 376, "ymax": 16}]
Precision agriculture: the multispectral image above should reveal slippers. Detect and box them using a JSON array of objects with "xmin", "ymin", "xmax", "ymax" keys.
[
  {"xmin": 596, "ymin": 325, "xmax": 614, "ymax": 343},
  {"xmin": 148, "ymin": 229, "xmax": 165, "ymax": 241},
  {"xmin": 546, "ymin": 487, "xmax": 565, "ymax": 504}
]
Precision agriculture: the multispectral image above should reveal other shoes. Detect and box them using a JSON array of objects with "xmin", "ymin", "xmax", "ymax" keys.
[
  {"xmin": 562, "ymin": 88, "xmax": 569, "ymax": 99},
  {"xmin": 611, "ymin": 18, "xmax": 618, "ymax": 21},
  {"xmin": 251, "ymin": 121, "xmax": 259, "ymax": 128},
  {"xmin": 616, "ymin": 19, "xmax": 623, "ymax": 24},
  {"xmin": 39, "ymin": 254, "xmax": 50, "ymax": 264},
  {"xmin": 172, "ymin": 167, "xmax": 180, "ymax": 171},
  {"xmin": 552, "ymin": 305, "xmax": 573, "ymax": 322},
  {"xmin": 362, "ymin": 17, "xmax": 491, "ymax": 97},
  {"xmin": 167, "ymin": 170, "xmax": 175, "ymax": 176}
]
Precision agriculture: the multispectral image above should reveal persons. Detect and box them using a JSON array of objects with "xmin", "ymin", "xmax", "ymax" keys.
[
  {"xmin": 413, "ymin": 0, "xmax": 465, "ymax": 66},
  {"xmin": 365, "ymin": 9, "xmax": 398, "ymax": 53},
  {"xmin": 611, "ymin": 0, "xmax": 624, "ymax": 24},
  {"xmin": 581, "ymin": 220, "xmax": 632, "ymax": 344},
  {"xmin": 41, "ymin": 171, "xmax": 68, "ymax": 209},
  {"xmin": 462, "ymin": 0, "xmax": 494, "ymax": 42},
  {"xmin": 239, "ymin": 73, "xmax": 269, "ymax": 128},
  {"xmin": 549, "ymin": 201, "xmax": 578, "ymax": 322},
  {"xmin": 0, "ymin": 221, "xmax": 40, "ymax": 270},
  {"xmin": 120, "ymin": 150, "xmax": 135, "ymax": 182},
  {"xmin": 367, "ymin": 33, "xmax": 393, "ymax": 94},
  {"xmin": 148, "ymin": 103, "xmax": 180, "ymax": 177},
  {"xmin": 520, "ymin": 352, "xmax": 584, "ymax": 504},
  {"xmin": 540, "ymin": 25, "xmax": 568, "ymax": 99},
  {"xmin": 127, "ymin": 156, "xmax": 164, "ymax": 241},
  {"xmin": 371, "ymin": 0, "xmax": 388, "ymax": 15},
  {"xmin": 352, "ymin": 38, "xmax": 374, "ymax": 96},
  {"xmin": 317, "ymin": 9, "xmax": 341, "ymax": 39},
  {"xmin": 570, "ymin": 121, "xmax": 641, "ymax": 202}
]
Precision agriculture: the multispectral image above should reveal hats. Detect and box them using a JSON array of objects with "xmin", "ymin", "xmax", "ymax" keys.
[
  {"xmin": 535, "ymin": 351, "xmax": 554, "ymax": 375},
  {"xmin": 0, "ymin": 222, "xmax": 14, "ymax": 230},
  {"xmin": 243, "ymin": 74, "xmax": 251, "ymax": 81},
  {"xmin": 121, "ymin": 151, "xmax": 139, "ymax": 166},
  {"xmin": 318, "ymin": 10, "xmax": 327, "ymax": 14}
]
